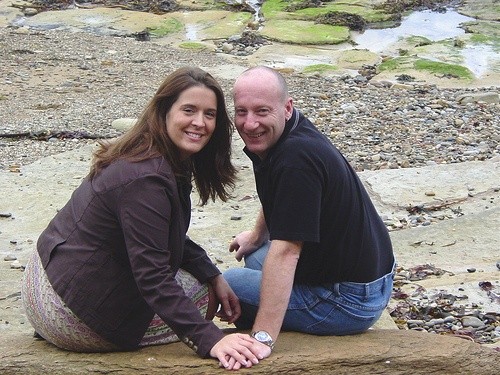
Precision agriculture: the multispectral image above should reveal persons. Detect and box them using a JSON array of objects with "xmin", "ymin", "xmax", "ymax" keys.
[
  {"xmin": 21, "ymin": 66, "xmax": 264, "ymax": 367},
  {"xmin": 219, "ymin": 65, "xmax": 397, "ymax": 371}
]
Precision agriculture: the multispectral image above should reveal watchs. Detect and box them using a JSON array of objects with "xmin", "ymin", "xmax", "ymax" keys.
[{"xmin": 249, "ymin": 330, "xmax": 275, "ymax": 351}]
